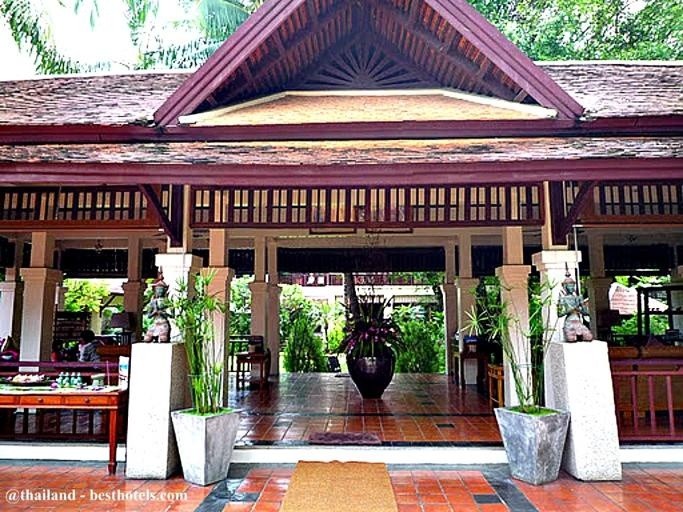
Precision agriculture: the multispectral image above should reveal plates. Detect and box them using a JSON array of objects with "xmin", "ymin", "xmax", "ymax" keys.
[{"xmin": 3, "ymin": 377, "xmax": 48, "ymax": 387}]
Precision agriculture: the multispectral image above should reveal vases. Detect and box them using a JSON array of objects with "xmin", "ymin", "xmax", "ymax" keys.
[{"xmin": 347, "ymin": 339, "xmax": 395, "ymax": 400}]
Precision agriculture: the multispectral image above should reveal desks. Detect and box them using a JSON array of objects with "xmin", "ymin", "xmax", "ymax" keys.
[
  {"xmin": 487, "ymin": 363, "xmax": 506, "ymax": 411},
  {"xmin": 0, "ymin": 380, "xmax": 127, "ymax": 477}
]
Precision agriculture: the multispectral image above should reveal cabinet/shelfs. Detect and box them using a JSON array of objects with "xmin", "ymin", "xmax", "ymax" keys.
[
  {"xmin": 234, "ymin": 351, "xmax": 271, "ymax": 392},
  {"xmin": 453, "ymin": 347, "xmax": 489, "ymax": 389}
]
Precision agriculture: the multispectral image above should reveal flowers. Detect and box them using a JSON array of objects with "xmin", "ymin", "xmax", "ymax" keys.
[{"xmin": 330, "ymin": 293, "xmax": 407, "ymax": 361}]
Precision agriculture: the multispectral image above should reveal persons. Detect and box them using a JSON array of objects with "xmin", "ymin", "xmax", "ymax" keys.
[
  {"xmin": 50, "ymin": 339, "xmax": 65, "ymax": 379},
  {"xmin": 556, "ymin": 279, "xmax": 592, "ymax": 342},
  {"xmin": 141, "ymin": 280, "xmax": 175, "ymax": 344},
  {"xmin": 77, "ymin": 330, "xmax": 103, "ymax": 373}
]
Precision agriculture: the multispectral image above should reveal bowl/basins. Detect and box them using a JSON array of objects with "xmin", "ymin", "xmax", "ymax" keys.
[{"xmin": 1, "ymin": 355, "xmax": 13, "ymax": 361}]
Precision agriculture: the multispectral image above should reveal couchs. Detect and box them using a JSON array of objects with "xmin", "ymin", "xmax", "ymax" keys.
[{"xmin": 608, "ymin": 345, "xmax": 683, "ymax": 425}]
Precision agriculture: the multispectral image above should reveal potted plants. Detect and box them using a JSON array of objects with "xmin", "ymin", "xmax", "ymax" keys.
[
  {"xmin": 154, "ymin": 269, "xmax": 243, "ymax": 487},
  {"xmin": 463, "ymin": 275, "xmax": 571, "ymax": 486}
]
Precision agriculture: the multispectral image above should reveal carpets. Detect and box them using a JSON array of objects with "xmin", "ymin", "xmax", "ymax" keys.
[{"xmin": 279, "ymin": 462, "xmax": 398, "ymax": 512}]
